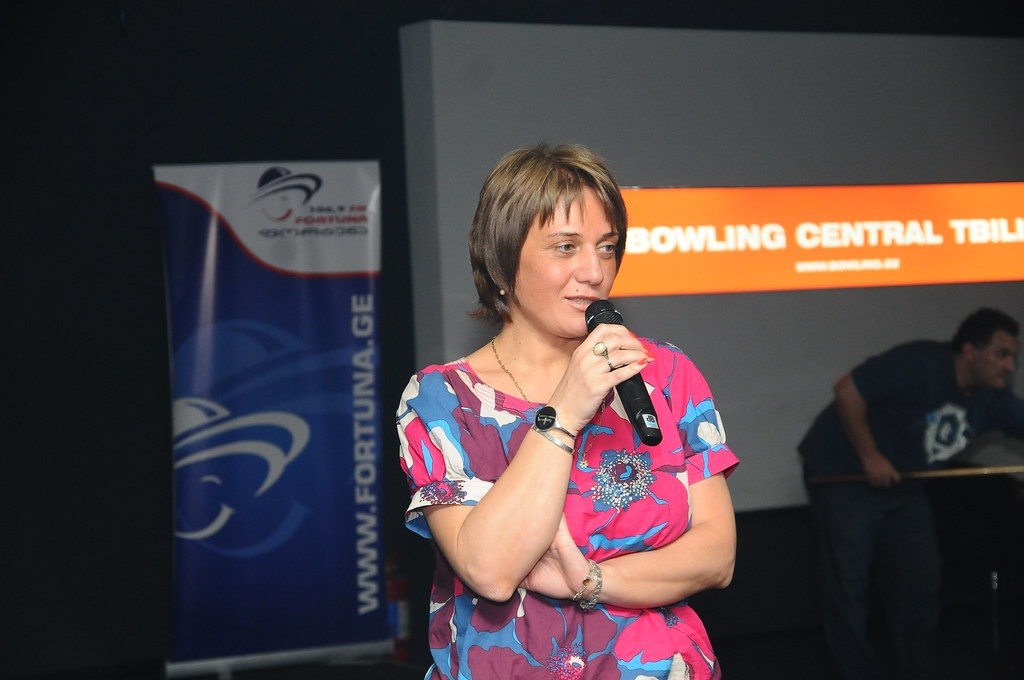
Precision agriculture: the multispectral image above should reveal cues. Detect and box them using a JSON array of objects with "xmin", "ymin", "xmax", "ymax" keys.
[{"xmin": 806, "ymin": 465, "xmax": 1024, "ymax": 482}]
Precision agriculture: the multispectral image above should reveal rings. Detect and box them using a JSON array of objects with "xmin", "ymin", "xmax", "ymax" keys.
[{"xmin": 594, "ymin": 342, "xmax": 616, "ymax": 371}]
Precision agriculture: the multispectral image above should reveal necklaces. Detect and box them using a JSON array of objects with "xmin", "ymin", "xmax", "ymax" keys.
[{"xmin": 492, "ymin": 336, "xmax": 605, "ymax": 469}]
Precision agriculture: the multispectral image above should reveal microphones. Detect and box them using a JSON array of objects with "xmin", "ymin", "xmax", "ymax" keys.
[{"xmin": 584, "ymin": 300, "xmax": 662, "ymax": 447}]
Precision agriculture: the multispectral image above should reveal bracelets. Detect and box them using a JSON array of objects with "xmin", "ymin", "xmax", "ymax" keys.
[
  {"xmin": 571, "ymin": 559, "xmax": 602, "ymax": 612},
  {"xmin": 529, "ymin": 406, "xmax": 576, "ymax": 457}
]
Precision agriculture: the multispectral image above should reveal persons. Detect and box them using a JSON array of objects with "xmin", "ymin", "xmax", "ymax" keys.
[
  {"xmin": 394, "ymin": 137, "xmax": 743, "ymax": 680},
  {"xmin": 799, "ymin": 308, "xmax": 1024, "ymax": 679}
]
